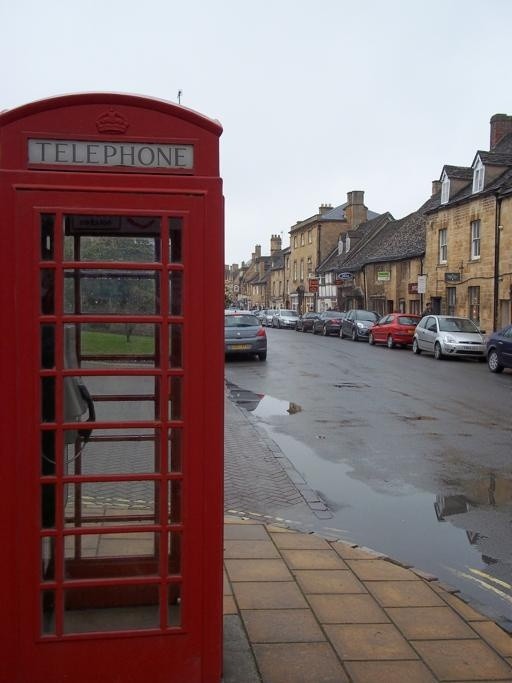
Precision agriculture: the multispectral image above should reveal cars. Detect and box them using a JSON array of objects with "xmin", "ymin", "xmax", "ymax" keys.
[
  {"xmin": 224, "ymin": 310, "xmax": 267, "ymax": 361},
  {"xmin": 256, "ymin": 310, "xmax": 512, "ymax": 373}
]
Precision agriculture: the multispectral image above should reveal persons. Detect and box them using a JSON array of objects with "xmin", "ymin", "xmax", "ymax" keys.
[{"xmin": 422, "ymin": 302, "xmax": 433, "ymax": 315}]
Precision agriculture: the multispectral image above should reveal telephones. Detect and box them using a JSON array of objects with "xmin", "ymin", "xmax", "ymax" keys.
[{"xmin": 37, "ymin": 320, "xmax": 95, "ymax": 443}]
